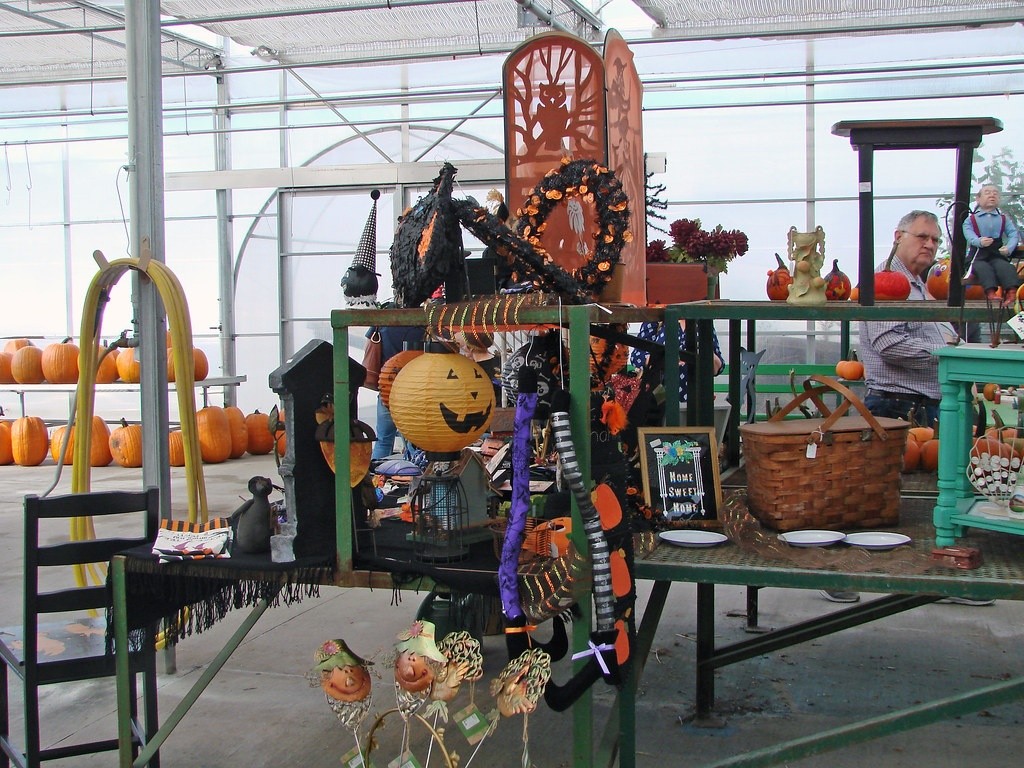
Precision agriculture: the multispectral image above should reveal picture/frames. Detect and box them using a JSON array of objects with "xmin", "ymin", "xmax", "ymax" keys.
[{"xmin": 637, "ymin": 426, "xmax": 725, "ymax": 529}]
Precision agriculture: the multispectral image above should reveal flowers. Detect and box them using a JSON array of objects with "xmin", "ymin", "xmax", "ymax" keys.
[{"xmin": 645, "ymin": 218, "xmax": 749, "ymax": 276}]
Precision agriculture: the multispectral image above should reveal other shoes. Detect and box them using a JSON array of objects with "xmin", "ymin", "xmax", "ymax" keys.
[
  {"xmin": 820, "ymin": 589, "xmax": 860, "ymax": 603},
  {"xmin": 936, "ymin": 596, "xmax": 997, "ymax": 605}
]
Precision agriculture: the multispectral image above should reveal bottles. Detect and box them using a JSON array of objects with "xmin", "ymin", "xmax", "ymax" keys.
[{"xmin": 659, "ymin": 529, "xmax": 727, "ymax": 547}]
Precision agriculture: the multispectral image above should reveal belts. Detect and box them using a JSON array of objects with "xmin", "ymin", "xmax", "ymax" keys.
[{"xmin": 868, "ymin": 389, "xmax": 938, "ymax": 405}]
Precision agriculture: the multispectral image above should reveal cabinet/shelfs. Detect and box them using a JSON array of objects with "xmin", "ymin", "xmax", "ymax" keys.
[{"xmin": 110, "ymin": 300, "xmax": 1024, "ymax": 768}]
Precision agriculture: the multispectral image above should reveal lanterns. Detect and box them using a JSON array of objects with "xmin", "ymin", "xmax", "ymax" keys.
[
  {"xmin": 388, "ymin": 341, "xmax": 496, "ymax": 462},
  {"xmin": 379, "ymin": 341, "xmax": 424, "ymax": 412},
  {"xmin": 591, "ymin": 327, "xmax": 632, "ymax": 382},
  {"xmin": 501, "ymin": 335, "xmax": 569, "ymax": 408}
]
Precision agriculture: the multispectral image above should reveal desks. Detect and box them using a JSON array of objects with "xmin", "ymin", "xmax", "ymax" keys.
[{"xmin": 935, "ymin": 342, "xmax": 1024, "ymax": 548}]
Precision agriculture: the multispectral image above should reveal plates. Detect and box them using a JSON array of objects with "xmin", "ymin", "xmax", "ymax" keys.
[
  {"xmin": 777, "ymin": 530, "xmax": 846, "ymax": 548},
  {"xmin": 841, "ymin": 532, "xmax": 912, "ymax": 551}
]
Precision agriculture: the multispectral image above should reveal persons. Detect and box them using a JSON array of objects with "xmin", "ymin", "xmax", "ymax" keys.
[
  {"xmin": 860, "ymin": 210, "xmax": 978, "ymax": 427},
  {"xmin": 962, "ymin": 183, "xmax": 1021, "ymax": 306},
  {"xmin": 366, "ymin": 296, "xmax": 499, "ymax": 461},
  {"xmin": 630, "ymin": 317, "xmax": 725, "ymax": 420}
]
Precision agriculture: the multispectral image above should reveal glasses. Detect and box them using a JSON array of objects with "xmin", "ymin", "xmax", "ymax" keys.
[{"xmin": 902, "ymin": 231, "xmax": 942, "ymax": 247}]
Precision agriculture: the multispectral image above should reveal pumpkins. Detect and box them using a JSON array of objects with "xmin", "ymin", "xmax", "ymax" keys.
[
  {"xmin": 0, "ymin": 329, "xmax": 208, "ymax": 384},
  {"xmin": 197, "ymin": 404, "xmax": 287, "ymax": 462},
  {"xmin": 766, "ymin": 253, "xmax": 793, "ymax": 301},
  {"xmin": 904, "ymin": 384, "xmax": 1024, "ymax": 473},
  {"xmin": 824, "ymin": 240, "xmax": 1023, "ymax": 301},
  {"xmin": 90, "ymin": 416, "xmax": 184, "ymax": 467},
  {"xmin": 0, "ymin": 416, "xmax": 76, "ymax": 465},
  {"xmin": 836, "ymin": 350, "xmax": 866, "ymax": 380}
]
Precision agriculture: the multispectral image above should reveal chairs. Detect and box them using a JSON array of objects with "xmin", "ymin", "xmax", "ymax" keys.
[{"xmin": 0, "ymin": 486, "xmax": 160, "ymax": 767}]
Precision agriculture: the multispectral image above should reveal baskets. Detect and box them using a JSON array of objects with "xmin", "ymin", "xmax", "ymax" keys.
[{"xmin": 738, "ymin": 376, "xmax": 912, "ymax": 532}]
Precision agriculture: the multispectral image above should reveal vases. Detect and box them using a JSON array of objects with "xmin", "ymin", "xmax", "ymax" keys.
[
  {"xmin": 645, "ymin": 264, "xmax": 720, "ymax": 302},
  {"xmin": 700, "ymin": 261, "xmax": 720, "ymax": 300}
]
